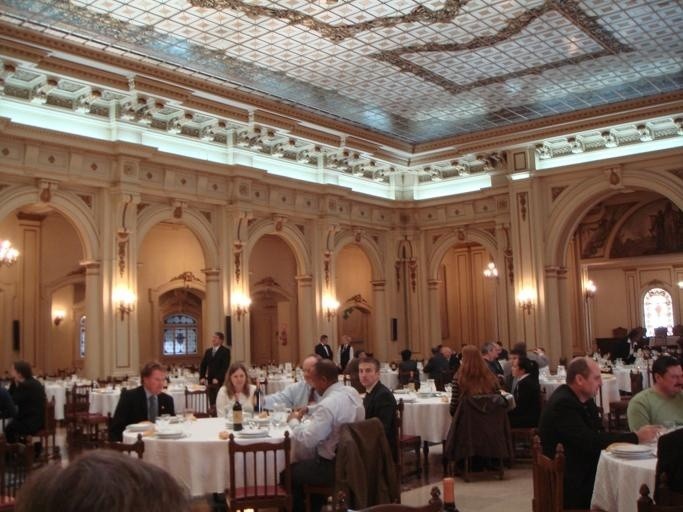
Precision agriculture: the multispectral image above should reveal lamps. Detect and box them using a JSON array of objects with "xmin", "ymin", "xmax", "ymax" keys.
[
  {"xmin": 114, "ymin": 283, "xmax": 135, "ymax": 321},
  {"xmin": 0, "ymin": 239, "xmax": 19, "ymax": 268},
  {"xmin": 232, "ymin": 291, "xmax": 250, "ymax": 320},
  {"xmin": 583, "ymin": 279, "xmax": 596, "ymax": 302},
  {"xmin": 517, "ymin": 287, "xmax": 535, "ymax": 316},
  {"xmin": 53, "ymin": 308, "xmax": 64, "ymax": 325},
  {"xmin": 322, "ymin": 293, "xmax": 340, "ymax": 322},
  {"xmin": 484, "ymin": 263, "xmax": 498, "ymax": 277}
]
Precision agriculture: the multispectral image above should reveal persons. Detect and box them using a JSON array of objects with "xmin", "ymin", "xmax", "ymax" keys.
[
  {"xmin": 216, "ymin": 363, "xmax": 256, "ymax": 418},
  {"xmin": 315, "ymin": 335, "xmax": 333, "ymax": 361},
  {"xmin": 508, "ymin": 356, "xmax": 540, "ymax": 427},
  {"xmin": 109, "ymin": 362, "xmax": 176, "ymax": 441},
  {"xmin": 278, "ymin": 359, "xmax": 365, "ymax": 512},
  {"xmin": 359, "ymin": 357, "xmax": 398, "ymax": 464},
  {"xmin": 5, "ymin": 361, "xmax": 46, "ymax": 466},
  {"xmin": 493, "ymin": 343, "xmax": 502, "ymax": 355},
  {"xmin": 538, "ymin": 357, "xmax": 658, "ymax": 510},
  {"xmin": 480, "ymin": 343, "xmax": 504, "ymax": 388},
  {"xmin": 349, "ymin": 349, "xmax": 366, "ymax": 394},
  {"xmin": 399, "ymin": 350, "xmax": 419, "ymax": 390},
  {"xmin": 449, "ymin": 349, "xmax": 458, "ymax": 367},
  {"xmin": 506, "ymin": 348, "xmax": 527, "ymax": 392},
  {"xmin": 337, "ymin": 335, "xmax": 354, "ymax": 375},
  {"xmin": 429, "ymin": 347, "xmax": 456, "ymax": 392},
  {"xmin": 423, "ymin": 348, "xmax": 440, "ymax": 373},
  {"xmin": 261, "ymin": 354, "xmax": 321, "ymax": 419},
  {"xmin": 0, "ymin": 378, "xmax": 18, "ymax": 433},
  {"xmin": 200, "ymin": 333, "xmax": 231, "ymax": 418},
  {"xmin": 449, "ymin": 345, "xmax": 501, "ymax": 417},
  {"xmin": 627, "ymin": 356, "xmax": 683, "ymax": 432},
  {"xmin": 497, "ymin": 342, "xmax": 509, "ymax": 361},
  {"xmin": 503, "ymin": 343, "xmax": 550, "ymax": 385},
  {"xmin": 612, "ymin": 329, "xmax": 642, "ymax": 365}
]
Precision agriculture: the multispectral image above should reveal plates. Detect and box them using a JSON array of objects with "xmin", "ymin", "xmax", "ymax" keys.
[
  {"xmin": 236, "ymin": 429, "xmax": 268, "ymax": 438},
  {"xmin": 607, "ymin": 444, "xmax": 654, "ymax": 458},
  {"xmin": 153, "ymin": 431, "xmax": 183, "ymax": 439}
]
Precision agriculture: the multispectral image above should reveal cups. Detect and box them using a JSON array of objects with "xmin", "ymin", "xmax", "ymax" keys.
[
  {"xmin": 154, "ymin": 413, "xmax": 170, "ymax": 431},
  {"xmin": 270, "ymin": 402, "xmax": 292, "ymax": 427}
]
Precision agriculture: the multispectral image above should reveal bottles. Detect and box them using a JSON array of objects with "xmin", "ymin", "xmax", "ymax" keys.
[
  {"xmin": 232, "ymin": 394, "xmax": 242, "ymax": 430},
  {"xmin": 174, "ymin": 367, "xmax": 178, "ymax": 379},
  {"xmin": 291, "ymin": 364, "xmax": 295, "ymax": 379},
  {"xmin": 408, "ymin": 371, "xmax": 416, "ymax": 392},
  {"xmin": 442, "ymin": 478, "xmax": 458, "ymax": 511},
  {"xmin": 252, "ymin": 378, "xmax": 263, "ymax": 413},
  {"xmin": 391, "ymin": 359, "xmax": 396, "ymax": 371}
]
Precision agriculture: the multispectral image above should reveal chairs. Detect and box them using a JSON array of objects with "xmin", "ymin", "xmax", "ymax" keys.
[
  {"xmin": 185, "ymin": 386, "xmax": 213, "ymax": 418},
  {"xmin": 99, "ymin": 431, "xmax": 143, "ymax": 458},
  {"xmin": 72, "ymin": 381, "xmax": 109, "ymax": 449},
  {"xmin": 0, "ymin": 433, "xmax": 33, "ymax": 499},
  {"xmin": 397, "ymin": 398, "xmax": 421, "ymax": 482},
  {"xmin": 638, "ymin": 428, "xmax": 683, "ymax": 512},
  {"xmin": 225, "ymin": 431, "xmax": 292, "ymax": 511},
  {"xmin": 532, "ymin": 435, "xmax": 563, "ymax": 511},
  {"xmin": 17, "ymin": 396, "xmax": 55, "ymax": 464},
  {"xmin": 64, "ymin": 388, "xmax": 94, "ymax": 446},
  {"xmin": 303, "ymin": 418, "xmax": 391, "ymax": 511},
  {"xmin": 609, "ymin": 370, "xmax": 643, "ymax": 434},
  {"xmin": 448, "ymin": 395, "xmax": 507, "ymax": 482}
]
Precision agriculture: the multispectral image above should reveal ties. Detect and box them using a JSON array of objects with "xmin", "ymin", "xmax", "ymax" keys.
[{"xmin": 148, "ymin": 394, "xmax": 157, "ymax": 424}]
